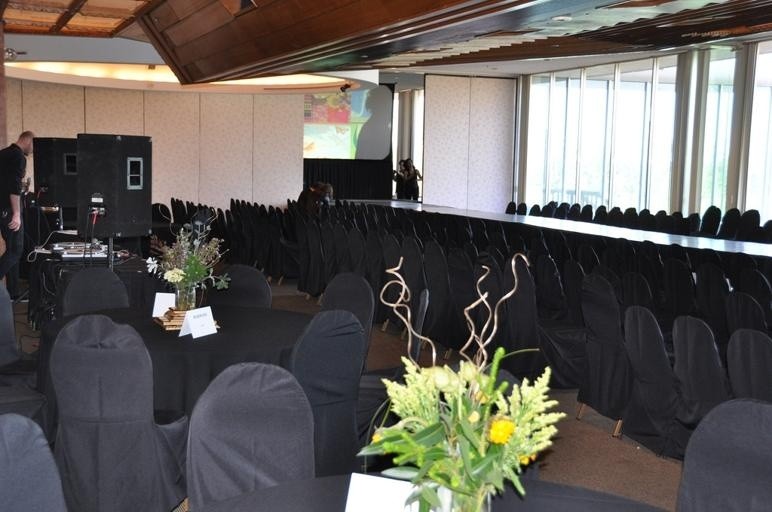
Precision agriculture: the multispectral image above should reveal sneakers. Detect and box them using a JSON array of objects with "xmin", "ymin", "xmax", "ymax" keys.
[{"xmin": 11, "ymin": 294, "xmax": 29, "ymax": 303}]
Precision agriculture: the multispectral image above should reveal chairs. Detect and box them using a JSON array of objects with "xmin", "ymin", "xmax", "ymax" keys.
[
  {"xmin": 424, "ymin": 216, "xmax": 762, "ymax": 467},
  {"xmin": 676, "ymin": 401, "xmax": 772, "ymax": 512},
  {"xmin": 0, "ymin": 262, "xmax": 372, "ymax": 512},
  {"xmin": 506, "ymin": 200, "xmax": 772, "ymax": 243},
  {"xmin": 170, "ymin": 198, "xmax": 441, "ymax": 303}
]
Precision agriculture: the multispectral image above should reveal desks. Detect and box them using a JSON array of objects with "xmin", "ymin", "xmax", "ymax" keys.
[{"xmin": 197, "ymin": 469, "xmax": 667, "ymax": 512}]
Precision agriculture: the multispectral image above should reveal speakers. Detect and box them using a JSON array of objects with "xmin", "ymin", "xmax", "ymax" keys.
[
  {"xmin": 75, "ymin": 132, "xmax": 154, "ymax": 239},
  {"xmin": 32, "ymin": 136, "xmax": 75, "ymax": 208}
]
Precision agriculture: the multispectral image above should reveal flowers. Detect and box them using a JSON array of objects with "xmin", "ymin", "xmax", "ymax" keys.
[{"xmin": 362, "ymin": 348, "xmax": 567, "ymax": 491}]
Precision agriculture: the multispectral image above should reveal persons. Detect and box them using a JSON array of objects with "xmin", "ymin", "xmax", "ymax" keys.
[
  {"xmin": 355, "ymin": 85, "xmax": 392, "ymax": 159},
  {"xmin": 404, "ymin": 158, "xmax": 423, "ymax": 201},
  {"xmin": 297, "ymin": 182, "xmax": 332, "ymax": 206},
  {"xmin": 393, "ymin": 160, "xmax": 407, "ymax": 200},
  {"xmin": 1, "ymin": 131, "xmax": 35, "ymax": 302}
]
